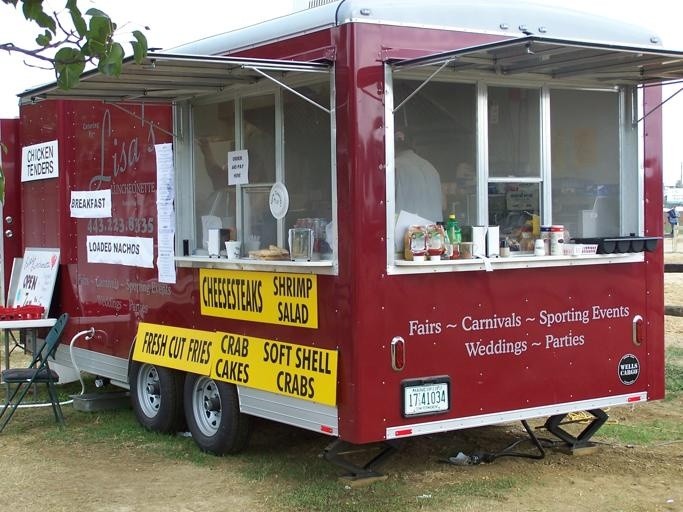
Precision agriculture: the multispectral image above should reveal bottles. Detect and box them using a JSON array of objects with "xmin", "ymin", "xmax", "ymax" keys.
[
  {"xmin": 291, "ymin": 218, "xmax": 333, "ymax": 263},
  {"xmin": 404, "ymin": 214, "xmax": 472, "ymax": 261},
  {"xmin": 534, "ymin": 225, "xmax": 564, "ymax": 257},
  {"xmin": 500, "ymin": 240, "xmax": 510, "ymax": 257}
]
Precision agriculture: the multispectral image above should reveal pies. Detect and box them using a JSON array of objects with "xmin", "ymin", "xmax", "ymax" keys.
[{"xmin": 260, "ymin": 247, "xmax": 290, "ymax": 260}]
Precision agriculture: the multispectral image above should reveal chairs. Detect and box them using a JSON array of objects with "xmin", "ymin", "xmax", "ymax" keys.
[{"xmin": 0, "ymin": 310, "xmax": 68, "ymax": 435}]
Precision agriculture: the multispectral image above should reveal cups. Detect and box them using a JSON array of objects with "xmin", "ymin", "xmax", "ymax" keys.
[{"xmin": 225, "ymin": 241, "xmax": 241, "ymax": 260}]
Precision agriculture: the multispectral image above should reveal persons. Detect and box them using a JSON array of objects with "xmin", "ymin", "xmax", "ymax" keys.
[
  {"xmin": 393, "ymin": 125, "xmax": 448, "ymax": 231},
  {"xmin": 668, "ymin": 205, "xmax": 680, "ymax": 234}
]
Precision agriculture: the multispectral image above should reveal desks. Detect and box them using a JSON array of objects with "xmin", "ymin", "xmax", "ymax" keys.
[{"xmin": 0, "ymin": 318, "xmax": 58, "ymax": 404}]
